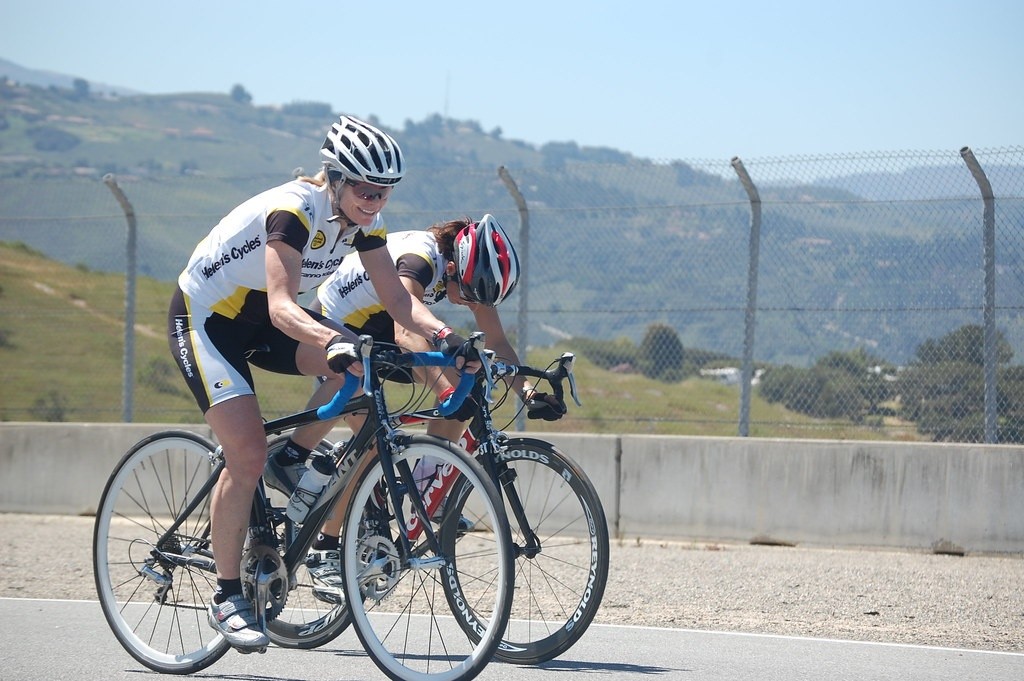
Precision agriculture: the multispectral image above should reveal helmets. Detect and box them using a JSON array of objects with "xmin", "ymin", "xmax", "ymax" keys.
[
  {"xmin": 319, "ymin": 115, "xmax": 403, "ymax": 186},
  {"xmin": 454, "ymin": 214, "xmax": 520, "ymax": 308}
]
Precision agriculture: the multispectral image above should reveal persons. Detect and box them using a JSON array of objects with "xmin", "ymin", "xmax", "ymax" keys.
[
  {"xmin": 166, "ymin": 115, "xmax": 483, "ymax": 649},
  {"xmin": 307, "ymin": 213, "xmax": 568, "ymax": 605}
]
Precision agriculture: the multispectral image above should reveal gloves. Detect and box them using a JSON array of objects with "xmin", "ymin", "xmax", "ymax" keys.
[
  {"xmin": 528, "ymin": 392, "xmax": 566, "ymax": 422},
  {"xmin": 440, "ymin": 386, "xmax": 478, "ymax": 422}
]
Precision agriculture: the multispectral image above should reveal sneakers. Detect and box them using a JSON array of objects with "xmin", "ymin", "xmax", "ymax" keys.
[
  {"xmin": 306, "ymin": 546, "xmax": 345, "ymax": 604},
  {"xmin": 432, "ymin": 497, "xmax": 475, "ymax": 531},
  {"xmin": 263, "ymin": 456, "xmax": 309, "ymax": 498},
  {"xmin": 207, "ymin": 591, "xmax": 270, "ymax": 646}
]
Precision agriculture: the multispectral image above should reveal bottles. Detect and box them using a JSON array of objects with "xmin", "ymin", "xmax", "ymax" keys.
[
  {"xmin": 413, "ymin": 451, "xmax": 445, "ymax": 494},
  {"xmin": 285, "ymin": 456, "xmax": 335, "ymax": 523}
]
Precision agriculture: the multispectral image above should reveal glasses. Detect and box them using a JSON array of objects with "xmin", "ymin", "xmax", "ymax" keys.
[
  {"xmin": 452, "ymin": 252, "xmax": 480, "ymax": 304},
  {"xmin": 353, "ymin": 182, "xmax": 392, "ymax": 200}
]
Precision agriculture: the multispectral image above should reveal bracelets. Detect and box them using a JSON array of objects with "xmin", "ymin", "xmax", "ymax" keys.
[{"xmin": 432, "ymin": 324, "xmax": 451, "ymax": 346}]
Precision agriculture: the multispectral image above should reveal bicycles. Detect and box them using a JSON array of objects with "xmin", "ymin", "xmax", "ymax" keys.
[
  {"xmin": 92, "ymin": 331, "xmax": 516, "ymax": 681},
  {"xmin": 264, "ymin": 352, "xmax": 610, "ymax": 666}
]
